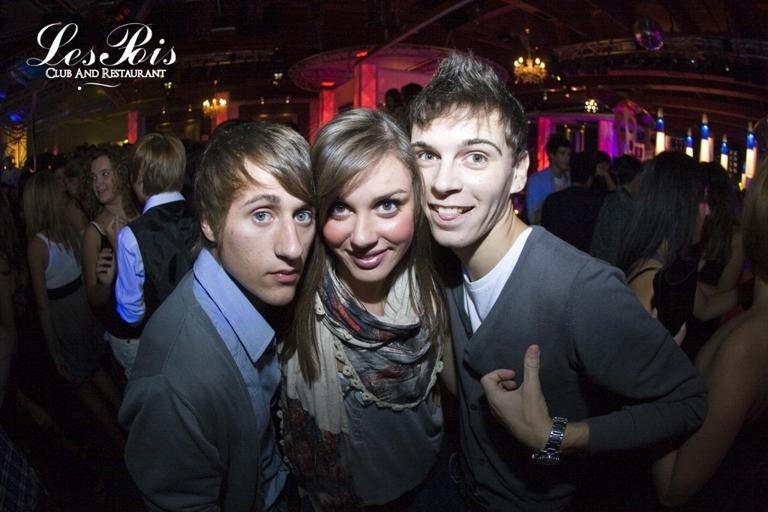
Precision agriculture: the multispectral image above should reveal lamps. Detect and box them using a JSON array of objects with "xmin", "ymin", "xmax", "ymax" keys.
[
  {"xmin": 510, "ymin": 23, "xmax": 548, "ymax": 85},
  {"xmin": 201, "ymin": 61, "xmax": 227, "ymax": 121}
]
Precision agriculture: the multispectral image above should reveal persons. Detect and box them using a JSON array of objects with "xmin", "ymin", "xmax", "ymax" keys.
[
  {"xmin": 111, "ymin": 133, "xmax": 209, "ymax": 324},
  {"xmin": 118, "ymin": 120, "xmax": 317, "ymax": 512},
  {"xmin": 693, "ymin": 163, "xmax": 752, "ymax": 359},
  {"xmin": 281, "ymin": 108, "xmax": 458, "ymax": 512},
  {"xmin": 541, "ymin": 153, "xmax": 616, "ymax": 257},
  {"xmin": 22, "ymin": 169, "xmax": 128, "ymax": 462},
  {"xmin": 83, "ymin": 144, "xmax": 160, "ymax": 380},
  {"xmin": 525, "ymin": 135, "xmax": 588, "ymax": 224},
  {"xmin": 407, "ymin": 50, "xmax": 707, "ymax": 510},
  {"xmin": 619, "ymin": 150, "xmax": 754, "ymax": 347},
  {"xmin": 0, "ymin": 141, "xmax": 131, "ymax": 187},
  {"xmin": 645, "ymin": 157, "xmax": 768, "ymax": 508},
  {"xmin": 591, "ymin": 151, "xmax": 616, "ymax": 194},
  {"xmin": 590, "ymin": 153, "xmax": 643, "ymax": 269}
]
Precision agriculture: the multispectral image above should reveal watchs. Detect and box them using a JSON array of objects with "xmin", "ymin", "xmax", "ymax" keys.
[{"xmin": 532, "ymin": 416, "xmax": 569, "ymax": 467}]
